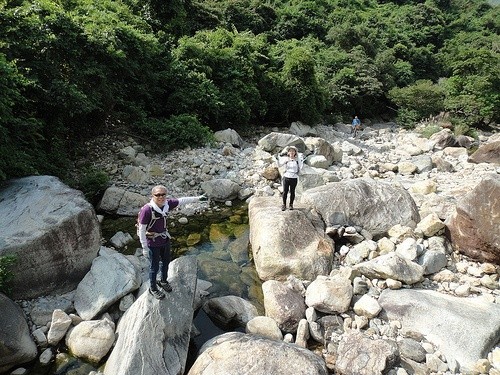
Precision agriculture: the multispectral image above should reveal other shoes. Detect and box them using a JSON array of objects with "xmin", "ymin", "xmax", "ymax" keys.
[
  {"xmin": 288, "ymin": 203, "xmax": 293, "ymax": 210},
  {"xmin": 281, "ymin": 204, "xmax": 286, "ymax": 211}
]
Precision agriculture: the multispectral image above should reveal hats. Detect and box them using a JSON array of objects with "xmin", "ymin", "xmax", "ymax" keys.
[{"xmin": 286, "ymin": 146, "xmax": 297, "ymax": 155}]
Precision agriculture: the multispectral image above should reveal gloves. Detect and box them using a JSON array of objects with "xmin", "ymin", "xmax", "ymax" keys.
[
  {"xmin": 199, "ymin": 192, "xmax": 210, "ymax": 201},
  {"xmin": 143, "ymin": 246, "xmax": 153, "ymax": 259}
]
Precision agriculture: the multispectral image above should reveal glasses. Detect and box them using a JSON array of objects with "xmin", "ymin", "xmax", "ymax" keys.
[{"xmin": 153, "ymin": 193, "xmax": 166, "ymax": 198}]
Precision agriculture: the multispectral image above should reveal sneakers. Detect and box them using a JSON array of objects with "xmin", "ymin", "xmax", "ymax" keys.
[
  {"xmin": 159, "ymin": 281, "xmax": 172, "ymax": 293},
  {"xmin": 149, "ymin": 287, "xmax": 165, "ymax": 299}
]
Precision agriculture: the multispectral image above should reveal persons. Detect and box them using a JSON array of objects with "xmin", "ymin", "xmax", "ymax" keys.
[
  {"xmin": 138, "ymin": 186, "xmax": 198, "ymax": 299},
  {"xmin": 352, "ymin": 116, "xmax": 360, "ymax": 138},
  {"xmin": 278, "ymin": 146, "xmax": 302, "ymax": 210}
]
